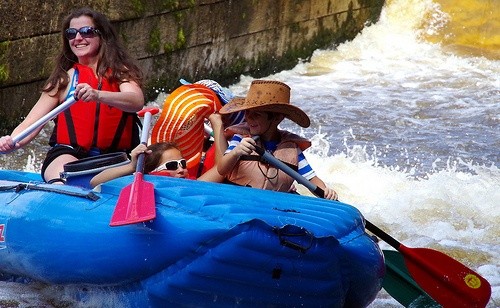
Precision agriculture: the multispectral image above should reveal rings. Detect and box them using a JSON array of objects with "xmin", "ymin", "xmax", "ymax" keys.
[{"xmin": 84, "ymin": 88, "xmax": 87, "ymax": 92}]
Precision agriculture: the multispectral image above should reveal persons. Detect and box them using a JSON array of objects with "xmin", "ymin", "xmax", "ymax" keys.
[
  {"xmin": 217, "ymin": 80, "xmax": 338, "ymax": 202},
  {"xmin": 180, "ymin": 78, "xmax": 234, "ymax": 128},
  {"xmin": 89, "ymin": 113, "xmax": 227, "ymax": 187},
  {"xmin": 0, "ymin": 8, "xmax": 144, "ymax": 187}
]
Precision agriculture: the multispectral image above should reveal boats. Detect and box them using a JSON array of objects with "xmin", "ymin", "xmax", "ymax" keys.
[{"xmin": 0, "ymin": 158, "xmax": 386, "ymax": 308}]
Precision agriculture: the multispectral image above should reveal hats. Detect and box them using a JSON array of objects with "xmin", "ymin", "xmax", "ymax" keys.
[{"xmin": 219, "ymin": 80, "xmax": 310, "ymax": 128}]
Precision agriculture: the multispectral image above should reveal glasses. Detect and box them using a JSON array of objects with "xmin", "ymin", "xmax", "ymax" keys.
[
  {"xmin": 151, "ymin": 159, "xmax": 187, "ymax": 173},
  {"xmin": 65, "ymin": 26, "xmax": 103, "ymax": 39}
]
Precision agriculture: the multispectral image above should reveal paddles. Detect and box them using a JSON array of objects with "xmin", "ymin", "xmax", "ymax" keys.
[
  {"xmin": 381, "ymin": 249, "xmax": 444, "ymax": 308},
  {"xmin": 248, "ymin": 142, "xmax": 493, "ymax": 308},
  {"xmin": 12, "ymin": 86, "xmax": 80, "ymax": 146},
  {"xmin": 109, "ymin": 107, "xmax": 159, "ymax": 228}
]
